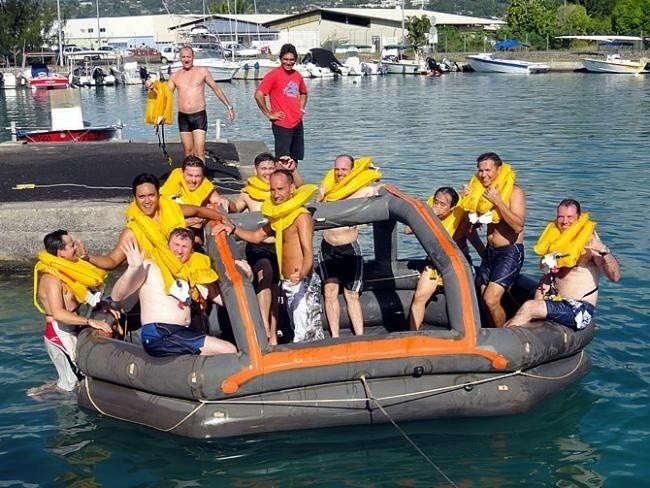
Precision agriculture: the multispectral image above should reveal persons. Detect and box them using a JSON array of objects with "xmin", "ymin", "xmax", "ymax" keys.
[
  {"xmin": 462, "ymin": 152, "xmax": 526, "ymax": 329},
  {"xmin": 146, "ymin": 48, "xmax": 235, "ymax": 157},
  {"xmin": 503, "ymin": 199, "xmax": 621, "ymax": 329},
  {"xmin": 254, "ymin": 44, "xmax": 308, "ymax": 168},
  {"xmin": 26, "ymin": 229, "xmax": 107, "ymax": 403},
  {"xmin": 316, "ymin": 154, "xmax": 385, "ymax": 338},
  {"xmin": 405, "ymin": 187, "xmax": 485, "ymax": 331}
]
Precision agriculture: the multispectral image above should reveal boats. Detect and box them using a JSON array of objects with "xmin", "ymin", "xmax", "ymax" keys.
[
  {"xmin": 3, "ymin": 105, "xmax": 121, "ymax": 143},
  {"xmin": 580, "ymin": 41, "xmax": 648, "ymax": 73},
  {"xmin": 465, "ymin": 40, "xmax": 551, "ymax": 73},
  {"xmin": 66, "ymin": 184, "xmax": 598, "ymax": 441}
]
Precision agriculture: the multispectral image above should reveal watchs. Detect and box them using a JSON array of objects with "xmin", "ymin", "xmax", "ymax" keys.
[{"xmin": 600, "ymin": 246, "xmax": 611, "ymax": 257}]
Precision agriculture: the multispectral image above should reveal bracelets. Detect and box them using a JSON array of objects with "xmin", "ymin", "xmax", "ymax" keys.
[
  {"xmin": 301, "ymin": 110, "xmax": 306, "ymax": 113},
  {"xmin": 227, "ymin": 106, "xmax": 233, "ymax": 110}
]
